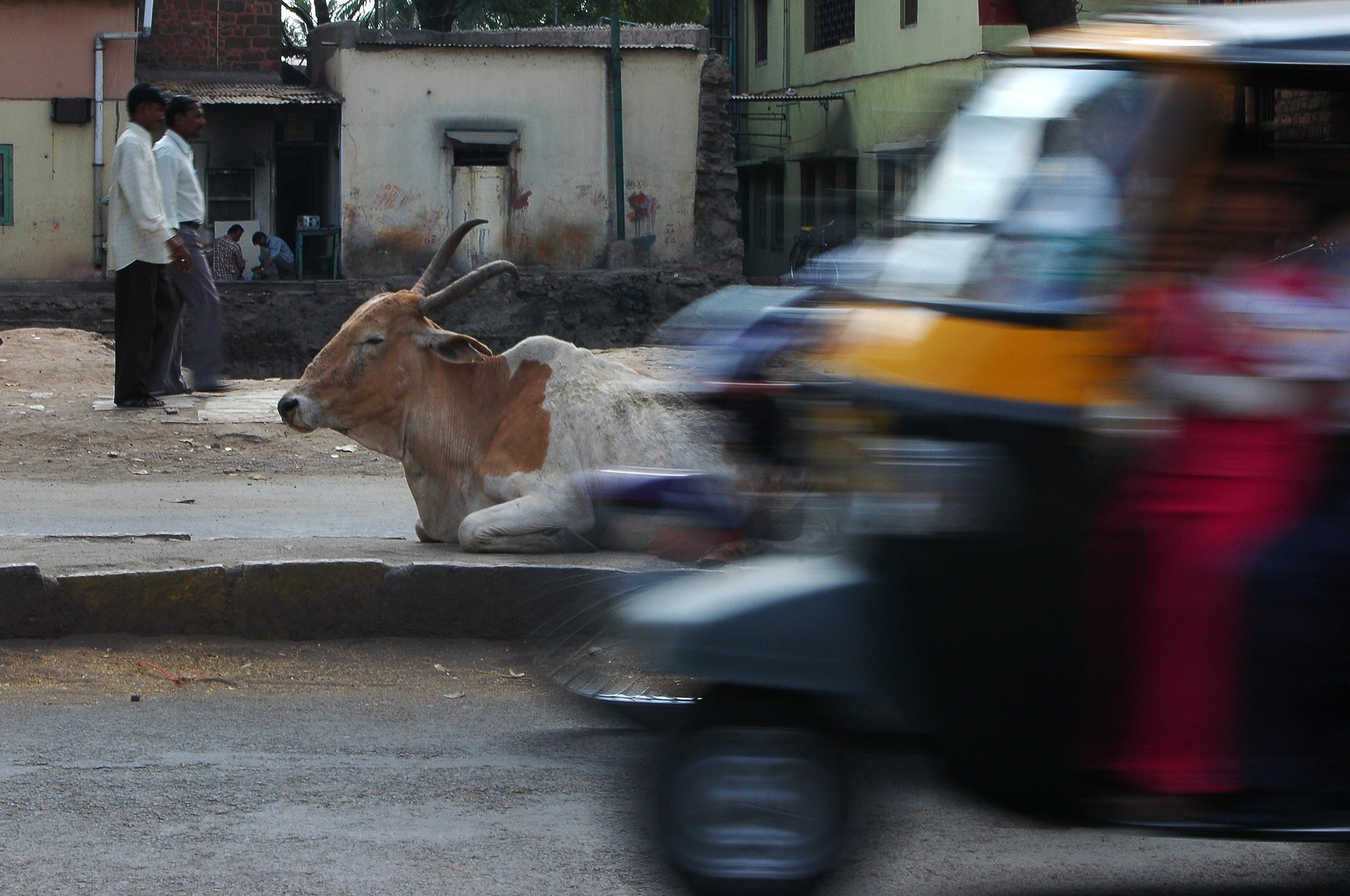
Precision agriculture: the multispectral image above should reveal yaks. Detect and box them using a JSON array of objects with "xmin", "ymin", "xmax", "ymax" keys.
[{"xmin": 274, "ymin": 217, "xmax": 731, "ymax": 550}]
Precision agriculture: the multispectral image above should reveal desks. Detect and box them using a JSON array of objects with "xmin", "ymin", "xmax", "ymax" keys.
[{"xmin": 297, "ymin": 226, "xmax": 340, "ymax": 280}]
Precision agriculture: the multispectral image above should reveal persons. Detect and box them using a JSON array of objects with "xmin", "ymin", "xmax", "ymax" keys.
[
  {"xmin": 105, "ymin": 81, "xmax": 234, "ymax": 410},
  {"xmin": 212, "ymin": 224, "xmax": 295, "ymax": 283}
]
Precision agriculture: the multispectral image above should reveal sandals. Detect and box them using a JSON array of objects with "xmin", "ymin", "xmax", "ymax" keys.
[{"xmin": 120, "ymin": 393, "xmax": 164, "ymax": 408}]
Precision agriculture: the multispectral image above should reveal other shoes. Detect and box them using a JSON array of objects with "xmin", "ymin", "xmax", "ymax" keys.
[
  {"xmin": 197, "ymin": 384, "xmax": 234, "ymax": 392},
  {"xmin": 185, "ymin": 386, "xmax": 194, "ymax": 394}
]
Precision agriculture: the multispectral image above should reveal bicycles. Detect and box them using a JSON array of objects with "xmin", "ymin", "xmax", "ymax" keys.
[{"xmin": 789, "ymin": 217, "xmax": 844, "ymax": 291}]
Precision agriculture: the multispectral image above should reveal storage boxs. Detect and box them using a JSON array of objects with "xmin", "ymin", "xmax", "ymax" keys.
[{"xmin": 297, "ymin": 216, "xmax": 321, "ymax": 230}]
